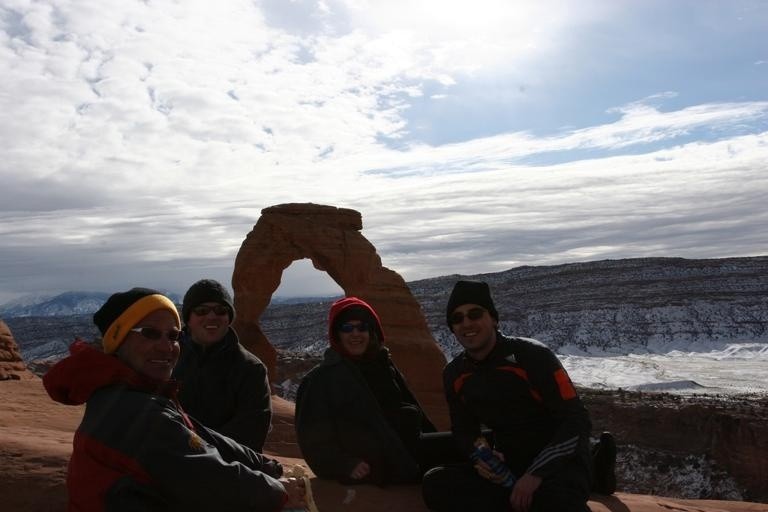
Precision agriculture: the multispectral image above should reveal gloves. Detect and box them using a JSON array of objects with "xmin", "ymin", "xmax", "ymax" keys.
[{"xmin": 350, "ymin": 462, "xmax": 370, "ymax": 481}]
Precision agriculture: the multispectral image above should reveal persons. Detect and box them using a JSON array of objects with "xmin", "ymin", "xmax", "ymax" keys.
[
  {"xmin": 296, "ymin": 297, "xmax": 616, "ymax": 496},
  {"xmin": 172, "ymin": 280, "xmax": 271, "ymax": 452},
  {"xmin": 423, "ymin": 281, "xmax": 590, "ymax": 511},
  {"xmin": 43, "ymin": 287, "xmax": 307, "ymax": 510}
]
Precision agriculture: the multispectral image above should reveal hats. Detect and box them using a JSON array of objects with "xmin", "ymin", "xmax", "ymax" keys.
[
  {"xmin": 446, "ymin": 282, "xmax": 499, "ymax": 332},
  {"xmin": 93, "ymin": 287, "xmax": 181, "ymax": 354},
  {"xmin": 182, "ymin": 279, "xmax": 235, "ymax": 327}
]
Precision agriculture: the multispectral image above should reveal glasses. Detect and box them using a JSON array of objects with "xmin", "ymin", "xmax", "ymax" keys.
[
  {"xmin": 448, "ymin": 308, "xmax": 487, "ymax": 323},
  {"xmin": 130, "ymin": 326, "xmax": 180, "ymax": 341},
  {"xmin": 339, "ymin": 323, "xmax": 371, "ymax": 334},
  {"xmin": 192, "ymin": 305, "xmax": 230, "ymax": 316}
]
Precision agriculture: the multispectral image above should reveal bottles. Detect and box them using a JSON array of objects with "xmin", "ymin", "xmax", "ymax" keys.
[{"xmin": 477, "ymin": 448, "xmax": 516, "ymax": 493}]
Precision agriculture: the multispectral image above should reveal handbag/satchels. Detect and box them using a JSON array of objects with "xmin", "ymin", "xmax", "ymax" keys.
[{"xmin": 580, "ymin": 432, "xmax": 616, "ymax": 495}]
